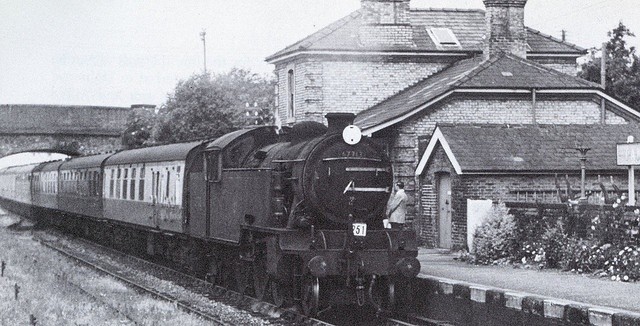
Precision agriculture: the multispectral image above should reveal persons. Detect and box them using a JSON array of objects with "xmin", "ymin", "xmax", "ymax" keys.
[{"xmin": 387, "ymin": 180, "xmax": 408, "ymax": 250}]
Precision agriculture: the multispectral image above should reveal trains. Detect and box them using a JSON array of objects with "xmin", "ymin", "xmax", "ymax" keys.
[{"xmin": 0, "ymin": 113, "xmax": 421, "ymax": 317}]
